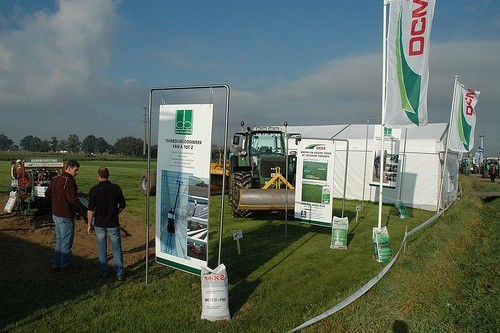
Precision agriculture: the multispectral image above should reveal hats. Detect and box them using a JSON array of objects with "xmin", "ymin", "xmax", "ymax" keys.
[{"xmin": 15, "ymin": 159, "xmax": 21, "ymax": 162}]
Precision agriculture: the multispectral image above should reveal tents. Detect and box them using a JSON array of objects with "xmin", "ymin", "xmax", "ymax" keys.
[{"xmin": 243, "ymin": 123, "xmax": 459, "ymax": 213}]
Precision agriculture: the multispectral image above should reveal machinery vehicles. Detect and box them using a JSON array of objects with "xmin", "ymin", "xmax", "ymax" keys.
[
  {"xmin": 140, "ymin": 161, "xmax": 229, "ymax": 196},
  {"xmin": 228, "ymin": 120, "xmax": 302, "ymax": 218}
]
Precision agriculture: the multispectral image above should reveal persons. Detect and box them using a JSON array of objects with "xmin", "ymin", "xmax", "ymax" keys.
[
  {"xmin": 49, "ymin": 161, "xmax": 88, "ymax": 272},
  {"xmin": 11, "ymin": 159, "xmax": 22, "ymax": 192},
  {"xmin": 87, "ymin": 167, "xmax": 126, "ymax": 280},
  {"xmin": 489, "ymin": 166, "xmax": 497, "ymax": 182}
]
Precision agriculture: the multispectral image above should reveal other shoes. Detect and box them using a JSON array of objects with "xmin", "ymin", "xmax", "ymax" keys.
[
  {"xmin": 53, "ymin": 266, "xmax": 64, "ymax": 273},
  {"xmin": 99, "ymin": 268, "xmax": 107, "ymax": 279},
  {"xmin": 117, "ymin": 277, "xmax": 125, "ymax": 281}
]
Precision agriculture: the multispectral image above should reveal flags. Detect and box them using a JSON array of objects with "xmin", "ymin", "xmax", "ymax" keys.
[
  {"xmin": 385, "ymin": 0, "xmax": 436, "ymax": 127},
  {"xmin": 448, "ymin": 82, "xmax": 480, "ymax": 152}
]
